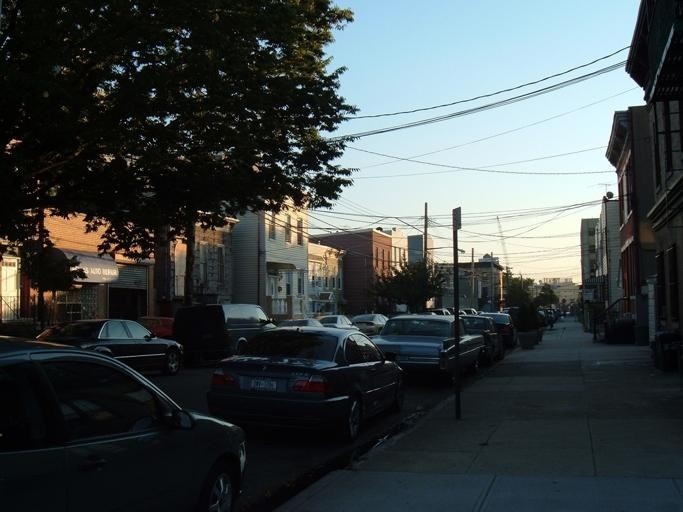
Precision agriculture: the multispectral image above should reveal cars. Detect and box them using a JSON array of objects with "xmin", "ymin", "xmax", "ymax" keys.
[
  {"xmin": 295, "ymin": 308, "xmax": 477, "ymax": 338},
  {"xmin": 217, "ymin": 313, "xmax": 423, "ymax": 445},
  {"xmin": 30, "ymin": 318, "xmax": 186, "ymax": 384},
  {"xmin": 368, "ymin": 304, "xmax": 528, "ymax": 387}
]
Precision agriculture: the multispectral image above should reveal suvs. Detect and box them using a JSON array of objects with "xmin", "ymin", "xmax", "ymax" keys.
[
  {"xmin": 179, "ymin": 292, "xmax": 283, "ymax": 363},
  {"xmin": 4, "ymin": 341, "xmax": 258, "ymax": 509}
]
